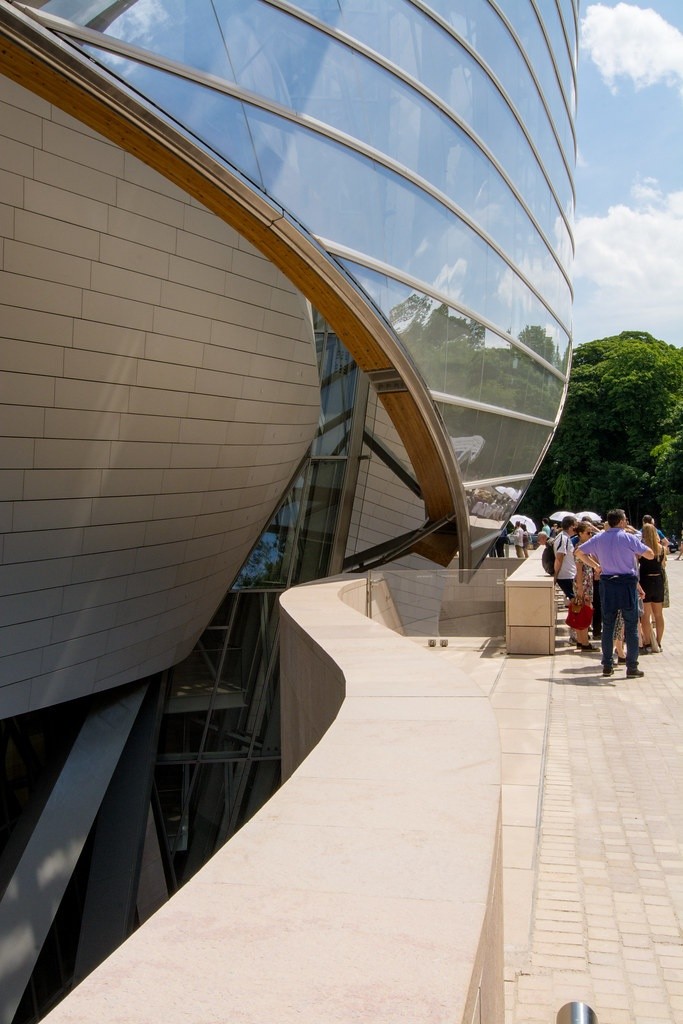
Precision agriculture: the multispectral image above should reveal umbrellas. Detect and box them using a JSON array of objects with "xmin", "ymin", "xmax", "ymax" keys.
[
  {"xmin": 549, "ymin": 511, "xmax": 576, "ymax": 521},
  {"xmin": 573, "ymin": 510, "xmax": 602, "ymax": 522},
  {"xmin": 510, "ymin": 513, "xmax": 537, "ymax": 534}
]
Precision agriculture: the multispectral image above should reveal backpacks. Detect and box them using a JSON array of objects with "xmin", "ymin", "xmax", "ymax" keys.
[{"xmin": 542, "ymin": 532, "xmax": 561, "ymax": 575}]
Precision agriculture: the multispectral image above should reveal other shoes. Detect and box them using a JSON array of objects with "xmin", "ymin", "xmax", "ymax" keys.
[
  {"xmin": 638, "ymin": 646, "xmax": 647, "ymax": 654},
  {"xmin": 569, "ymin": 638, "xmax": 578, "ymax": 646},
  {"xmin": 603, "ymin": 666, "xmax": 614, "ymax": 675},
  {"xmin": 576, "ymin": 642, "xmax": 582, "ymax": 649},
  {"xmin": 618, "ymin": 655, "xmax": 640, "ymax": 665},
  {"xmin": 643, "ymin": 643, "xmax": 652, "ymax": 653},
  {"xmin": 580, "ymin": 643, "xmax": 600, "ymax": 652},
  {"xmin": 627, "ymin": 669, "xmax": 644, "ymax": 678},
  {"xmin": 658, "ymin": 645, "xmax": 663, "ymax": 651},
  {"xmin": 592, "ymin": 633, "xmax": 601, "ymax": 640}
]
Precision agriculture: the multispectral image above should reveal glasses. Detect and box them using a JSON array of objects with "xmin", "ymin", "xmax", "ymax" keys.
[
  {"xmin": 583, "ymin": 531, "xmax": 593, "ymax": 535},
  {"xmin": 621, "ymin": 518, "xmax": 628, "ymax": 523}
]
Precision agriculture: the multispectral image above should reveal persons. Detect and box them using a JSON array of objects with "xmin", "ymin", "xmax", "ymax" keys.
[
  {"xmin": 537, "ymin": 509, "xmax": 669, "ymax": 678},
  {"xmin": 674, "ymin": 529, "xmax": 683, "ymax": 560},
  {"xmin": 541, "ymin": 517, "xmax": 551, "ymax": 538},
  {"xmin": 487, "ymin": 519, "xmax": 531, "ymax": 558}
]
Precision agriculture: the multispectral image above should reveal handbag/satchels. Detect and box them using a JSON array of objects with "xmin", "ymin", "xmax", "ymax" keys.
[{"xmin": 566, "ymin": 598, "xmax": 594, "ymax": 630}]
